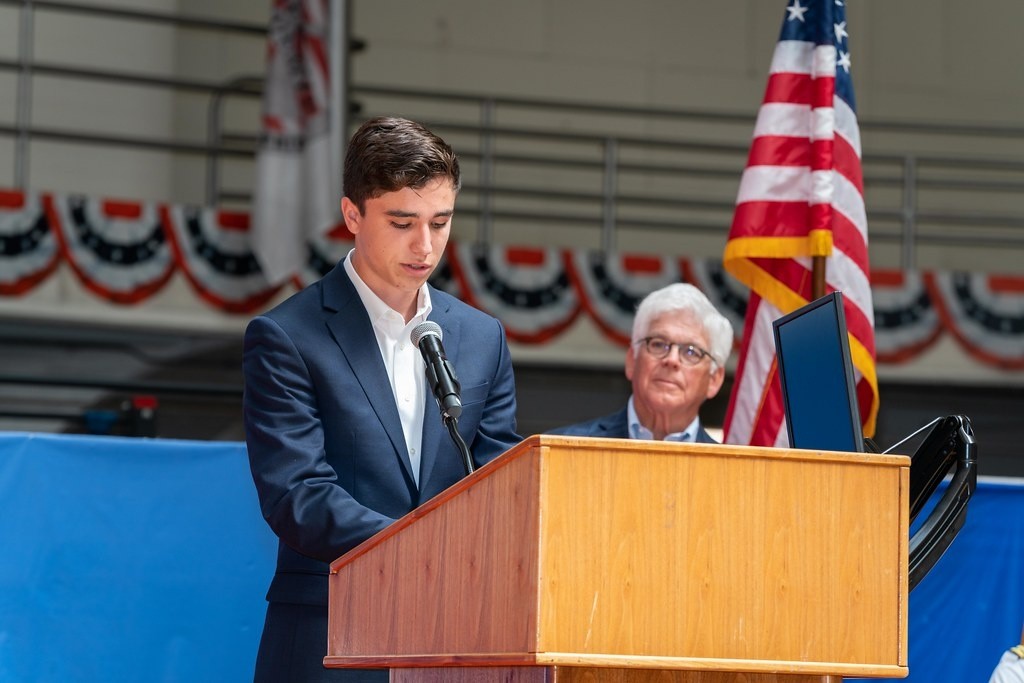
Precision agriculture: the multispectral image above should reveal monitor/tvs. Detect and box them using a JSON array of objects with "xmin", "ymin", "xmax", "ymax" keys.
[{"xmin": 772, "ymin": 290, "xmax": 864, "ymax": 452}]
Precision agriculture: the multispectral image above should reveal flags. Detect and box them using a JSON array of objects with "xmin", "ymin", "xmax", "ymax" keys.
[
  {"xmin": 723, "ymin": 0, "xmax": 878, "ymax": 458},
  {"xmin": 247, "ymin": 0, "xmax": 348, "ymax": 289}
]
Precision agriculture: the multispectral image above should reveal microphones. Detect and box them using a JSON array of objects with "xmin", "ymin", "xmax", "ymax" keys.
[{"xmin": 410, "ymin": 320, "xmax": 464, "ymax": 417}]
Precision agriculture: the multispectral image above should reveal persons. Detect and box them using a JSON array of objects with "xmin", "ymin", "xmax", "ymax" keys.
[
  {"xmin": 242, "ymin": 116, "xmax": 521, "ymax": 682},
  {"xmin": 543, "ymin": 284, "xmax": 733, "ymax": 446}
]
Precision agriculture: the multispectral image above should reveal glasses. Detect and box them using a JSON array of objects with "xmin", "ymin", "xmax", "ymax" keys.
[{"xmin": 638, "ymin": 335, "xmax": 717, "ymax": 365}]
